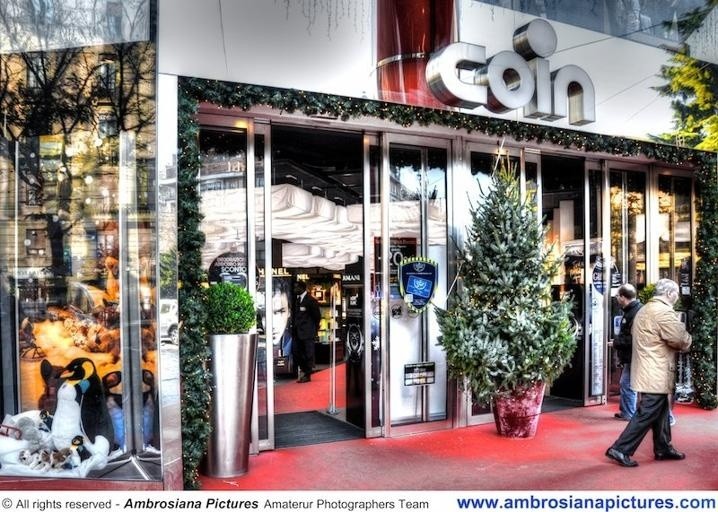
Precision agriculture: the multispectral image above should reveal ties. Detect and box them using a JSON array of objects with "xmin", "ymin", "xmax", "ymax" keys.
[{"xmin": 296, "ymin": 296, "xmax": 301, "ymax": 318}]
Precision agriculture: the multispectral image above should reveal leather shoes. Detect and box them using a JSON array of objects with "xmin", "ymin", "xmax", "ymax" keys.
[
  {"xmin": 605, "ymin": 447, "xmax": 639, "ymax": 468},
  {"xmin": 654, "ymin": 445, "xmax": 686, "ymax": 459},
  {"xmin": 296, "ymin": 373, "xmax": 311, "ymax": 383}
]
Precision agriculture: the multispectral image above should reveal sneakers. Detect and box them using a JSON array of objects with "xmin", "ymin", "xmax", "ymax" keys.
[{"xmin": 615, "ymin": 412, "xmax": 630, "ymax": 420}]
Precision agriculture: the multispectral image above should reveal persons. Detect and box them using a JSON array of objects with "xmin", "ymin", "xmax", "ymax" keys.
[
  {"xmin": 6, "ymin": 276, "xmax": 28, "ymax": 339},
  {"xmin": 257, "ymin": 278, "xmax": 289, "ymax": 345},
  {"xmin": 292, "ymin": 281, "xmax": 321, "ymax": 382},
  {"xmin": 603, "ymin": 279, "xmax": 692, "ymax": 467},
  {"xmin": 607, "ymin": 283, "xmax": 644, "ymax": 419}
]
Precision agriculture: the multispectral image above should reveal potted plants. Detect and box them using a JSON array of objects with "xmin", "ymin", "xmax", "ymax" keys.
[
  {"xmin": 205, "ymin": 281, "xmax": 258, "ymax": 480},
  {"xmin": 431, "ymin": 149, "xmax": 580, "ymax": 436}
]
